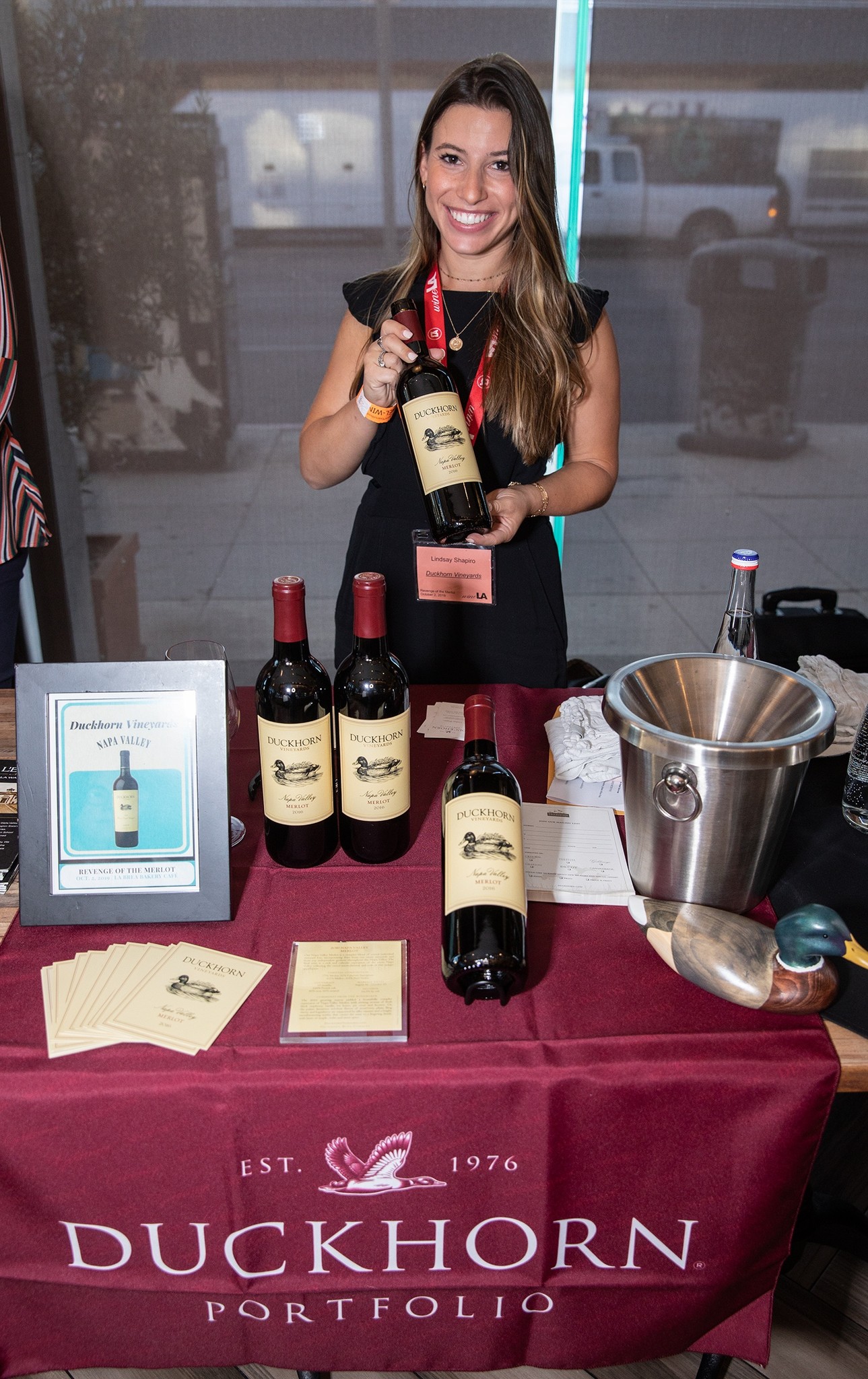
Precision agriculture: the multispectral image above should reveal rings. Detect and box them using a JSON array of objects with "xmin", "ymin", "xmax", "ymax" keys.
[
  {"xmin": 378, "ymin": 336, "xmax": 388, "ymax": 352},
  {"xmin": 378, "ymin": 350, "xmax": 388, "ymax": 368}
]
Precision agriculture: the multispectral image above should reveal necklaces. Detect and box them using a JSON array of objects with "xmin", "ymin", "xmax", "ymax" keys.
[
  {"xmin": 440, "ymin": 284, "xmax": 502, "ymax": 351},
  {"xmin": 438, "ymin": 266, "xmax": 510, "ymax": 282}
]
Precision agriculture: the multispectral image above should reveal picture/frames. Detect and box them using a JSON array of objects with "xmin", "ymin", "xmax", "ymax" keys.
[{"xmin": 15, "ymin": 659, "xmax": 233, "ymax": 925}]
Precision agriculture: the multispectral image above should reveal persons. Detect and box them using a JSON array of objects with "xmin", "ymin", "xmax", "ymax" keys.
[{"xmin": 299, "ymin": 54, "xmax": 620, "ymax": 690}]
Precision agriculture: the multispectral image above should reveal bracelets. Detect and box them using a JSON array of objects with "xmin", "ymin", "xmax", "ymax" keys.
[
  {"xmin": 356, "ymin": 384, "xmax": 397, "ymax": 424},
  {"xmin": 508, "ymin": 481, "xmax": 549, "ymax": 518}
]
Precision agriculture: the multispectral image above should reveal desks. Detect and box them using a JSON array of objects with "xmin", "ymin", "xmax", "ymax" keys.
[{"xmin": 1, "ymin": 678, "xmax": 867, "ymax": 1379}]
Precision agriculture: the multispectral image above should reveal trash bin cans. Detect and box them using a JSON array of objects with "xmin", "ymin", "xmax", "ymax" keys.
[{"xmin": 671, "ymin": 237, "xmax": 831, "ymax": 464}]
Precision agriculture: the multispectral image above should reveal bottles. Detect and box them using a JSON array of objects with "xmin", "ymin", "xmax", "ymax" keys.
[
  {"xmin": 387, "ymin": 294, "xmax": 495, "ymax": 544},
  {"xmin": 710, "ymin": 550, "xmax": 760, "ymax": 659},
  {"xmin": 255, "ymin": 572, "xmax": 415, "ymax": 868},
  {"xmin": 840, "ymin": 703, "xmax": 868, "ymax": 835},
  {"xmin": 435, "ymin": 691, "xmax": 529, "ymax": 999},
  {"xmin": 109, "ymin": 750, "xmax": 141, "ymax": 850}
]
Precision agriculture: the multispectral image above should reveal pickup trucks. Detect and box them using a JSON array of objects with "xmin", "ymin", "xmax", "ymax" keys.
[{"xmin": 579, "ymin": 143, "xmax": 779, "ymax": 254}]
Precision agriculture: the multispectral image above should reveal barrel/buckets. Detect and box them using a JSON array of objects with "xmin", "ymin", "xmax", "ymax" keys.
[{"xmin": 601, "ymin": 651, "xmax": 839, "ymax": 914}]
